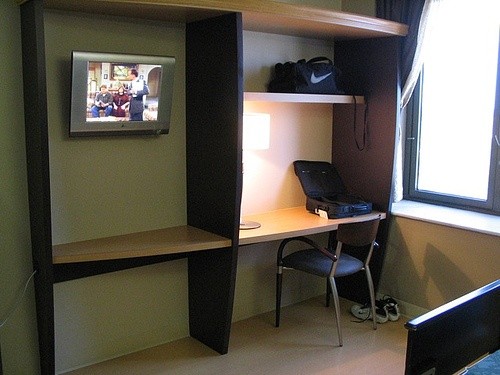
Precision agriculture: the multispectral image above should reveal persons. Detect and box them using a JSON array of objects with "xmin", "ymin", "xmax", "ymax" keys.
[
  {"xmin": 112, "ymin": 85, "xmax": 130, "ymax": 117},
  {"xmin": 127, "ymin": 68, "xmax": 150, "ymax": 121},
  {"xmin": 91, "ymin": 83, "xmax": 113, "ymax": 117}
]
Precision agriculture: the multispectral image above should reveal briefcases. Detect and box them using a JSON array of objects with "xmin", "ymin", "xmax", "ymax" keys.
[{"xmin": 293, "ymin": 159, "xmax": 373, "ymax": 219}]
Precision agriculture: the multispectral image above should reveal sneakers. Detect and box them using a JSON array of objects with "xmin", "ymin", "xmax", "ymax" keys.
[
  {"xmin": 378, "ymin": 295, "xmax": 400, "ymax": 323},
  {"xmin": 350, "ymin": 301, "xmax": 389, "ymax": 325}
]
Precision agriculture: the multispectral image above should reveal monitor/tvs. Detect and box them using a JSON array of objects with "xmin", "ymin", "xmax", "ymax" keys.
[{"xmin": 69, "ymin": 50, "xmax": 175, "ymax": 137}]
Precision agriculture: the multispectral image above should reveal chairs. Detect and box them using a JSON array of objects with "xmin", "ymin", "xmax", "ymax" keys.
[
  {"xmin": 403, "ymin": 278, "xmax": 500, "ymax": 375},
  {"xmin": 275, "ymin": 212, "xmax": 382, "ymax": 347}
]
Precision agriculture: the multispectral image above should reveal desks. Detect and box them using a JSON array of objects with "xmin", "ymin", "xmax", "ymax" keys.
[{"xmin": 460, "ymin": 348, "xmax": 500, "ymax": 374}]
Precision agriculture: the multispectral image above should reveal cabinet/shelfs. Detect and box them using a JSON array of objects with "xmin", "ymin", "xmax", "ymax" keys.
[
  {"xmin": 13, "ymin": 1, "xmax": 245, "ymax": 374},
  {"xmin": 225, "ymin": 1, "xmax": 409, "ymax": 356}
]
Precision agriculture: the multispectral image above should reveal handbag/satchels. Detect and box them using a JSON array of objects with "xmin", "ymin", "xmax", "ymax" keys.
[
  {"xmin": 267, "ymin": 55, "xmax": 338, "ymax": 94},
  {"xmin": 341, "ymin": 57, "xmax": 373, "ymax": 96}
]
